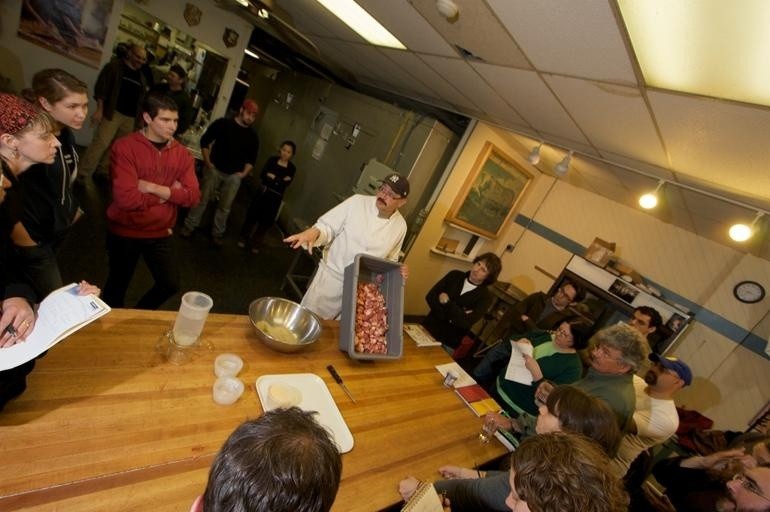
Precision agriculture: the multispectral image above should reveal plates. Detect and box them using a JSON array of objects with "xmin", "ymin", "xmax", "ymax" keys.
[{"xmin": 256, "ymin": 373, "xmax": 355, "ymax": 457}]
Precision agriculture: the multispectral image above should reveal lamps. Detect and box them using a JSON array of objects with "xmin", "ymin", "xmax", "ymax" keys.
[
  {"xmin": 554, "ymin": 151, "xmax": 574, "ymax": 176},
  {"xmin": 527, "ymin": 140, "xmax": 544, "ymax": 164},
  {"xmin": 729, "ymin": 211, "xmax": 764, "ymax": 243},
  {"xmin": 638, "ymin": 180, "xmax": 664, "ymax": 209}
]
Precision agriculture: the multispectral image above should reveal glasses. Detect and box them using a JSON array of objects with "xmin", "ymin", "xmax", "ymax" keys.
[{"xmin": 734, "ymin": 474, "xmax": 770, "ymax": 502}]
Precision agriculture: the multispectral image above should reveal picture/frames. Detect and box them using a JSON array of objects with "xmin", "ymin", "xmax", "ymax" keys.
[{"xmin": 445, "ymin": 140, "xmax": 534, "ymax": 241}]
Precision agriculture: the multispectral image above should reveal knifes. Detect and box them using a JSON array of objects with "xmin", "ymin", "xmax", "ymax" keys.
[{"xmin": 327, "ymin": 362, "xmax": 357, "ymax": 406}]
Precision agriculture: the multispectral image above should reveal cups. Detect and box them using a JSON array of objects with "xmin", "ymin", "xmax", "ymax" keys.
[
  {"xmin": 478, "ymin": 415, "xmax": 500, "ymax": 445},
  {"xmin": 172, "ymin": 291, "xmax": 215, "ymax": 345},
  {"xmin": 442, "ymin": 368, "xmax": 459, "ymax": 388},
  {"xmin": 214, "ymin": 353, "xmax": 244, "ymax": 376},
  {"xmin": 166, "ymin": 330, "xmax": 197, "ymax": 365},
  {"xmin": 213, "ymin": 376, "xmax": 244, "ymax": 405}
]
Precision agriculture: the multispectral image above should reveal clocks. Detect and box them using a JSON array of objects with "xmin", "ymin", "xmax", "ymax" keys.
[{"xmin": 733, "ymin": 280, "xmax": 765, "ymax": 303}]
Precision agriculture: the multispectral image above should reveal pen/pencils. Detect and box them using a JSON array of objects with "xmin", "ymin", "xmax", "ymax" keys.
[
  {"xmin": 6, "ymin": 324, "xmax": 17, "ymax": 337},
  {"xmin": 441, "ymin": 489, "xmax": 447, "ymax": 508}
]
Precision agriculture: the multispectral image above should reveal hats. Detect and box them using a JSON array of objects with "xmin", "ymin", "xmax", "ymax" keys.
[
  {"xmin": 648, "ymin": 351, "xmax": 691, "ymax": 386},
  {"xmin": 377, "ymin": 174, "xmax": 409, "ymax": 197},
  {"xmin": 242, "ymin": 97, "xmax": 258, "ymax": 114}
]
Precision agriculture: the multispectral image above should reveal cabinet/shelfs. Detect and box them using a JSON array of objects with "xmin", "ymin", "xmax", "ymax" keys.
[{"xmin": 547, "ymin": 253, "xmax": 694, "ymax": 371}]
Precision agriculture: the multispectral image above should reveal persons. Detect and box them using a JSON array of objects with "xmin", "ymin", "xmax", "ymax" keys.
[
  {"xmin": 188, "ymin": 406, "xmax": 343, "ymax": 512},
  {"xmin": 398, "ymin": 251, "xmax": 770, "ymax": 512},
  {"xmin": 1, "ymin": 36, "xmax": 297, "ymax": 412},
  {"xmin": 282, "ymin": 174, "xmax": 410, "ymax": 321}
]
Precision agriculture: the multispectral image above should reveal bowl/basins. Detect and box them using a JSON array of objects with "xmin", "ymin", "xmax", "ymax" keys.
[{"xmin": 248, "ymin": 297, "xmax": 322, "ymax": 354}]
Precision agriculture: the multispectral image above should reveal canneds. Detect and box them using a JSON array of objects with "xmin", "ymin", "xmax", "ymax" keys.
[{"xmin": 443, "ymin": 370, "xmax": 458, "ymax": 388}]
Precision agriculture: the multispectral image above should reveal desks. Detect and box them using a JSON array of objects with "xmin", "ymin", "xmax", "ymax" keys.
[{"xmin": 0, "ymin": 309, "xmax": 511, "ymax": 512}]
